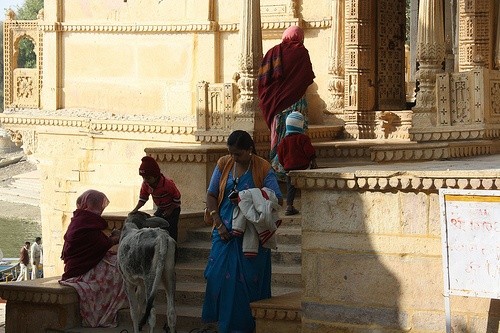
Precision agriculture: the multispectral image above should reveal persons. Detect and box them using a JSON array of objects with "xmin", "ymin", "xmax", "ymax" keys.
[
  {"xmin": 31, "ymin": 237, "xmax": 42, "ymax": 281},
  {"xmin": 132, "ymin": 156, "xmax": 182, "ymax": 264},
  {"xmin": 201, "ymin": 130, "xmax": 284, "ymax": 333},
  {"xmin": 277, "ymin": 111, "xmax": 319, "ymax": 216},
  {"xmin": 58, "ymin": 190, "xmax": 130, "ymax": 328},
  {"xmin": 16, "ymin": 242, "xmax": 30, "ymax": 281},
  {"xmin": 257, "ymin": 26, "xmax": 316, "ymax": 172}
]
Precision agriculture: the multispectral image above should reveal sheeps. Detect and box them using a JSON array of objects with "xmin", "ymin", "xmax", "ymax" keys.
[{"xmin": 117, "ymin": 211, "xmax": 176, "ymax": 333}]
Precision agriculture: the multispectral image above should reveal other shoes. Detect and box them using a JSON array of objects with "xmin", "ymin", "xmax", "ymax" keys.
[{"xmin": 285, "ymin": 209, "xmax": 299, "ymax": 215}]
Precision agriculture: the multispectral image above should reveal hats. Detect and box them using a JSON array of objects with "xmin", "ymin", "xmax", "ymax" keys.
[
  {"xmin": 285, "ymin": 110, "xmax": 304, "ymax": 134},
  {"xmin": 138, "ymin": 155, "xmax": 161, "ymax": 176}
]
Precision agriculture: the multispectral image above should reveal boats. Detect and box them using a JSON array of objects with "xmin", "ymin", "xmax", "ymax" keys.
[
  {"xmin": 0, "ymin": 127, "xmax": 24, "ymax": 168},
  {"xmin": 0, "ymin": 257, "xmax": 44, "ymax": 282}
]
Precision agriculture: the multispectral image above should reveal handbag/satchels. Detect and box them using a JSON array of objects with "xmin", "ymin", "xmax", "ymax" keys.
[{"xmin": 203, "ymin": 206, "xmax": 213, "ymax": 227}]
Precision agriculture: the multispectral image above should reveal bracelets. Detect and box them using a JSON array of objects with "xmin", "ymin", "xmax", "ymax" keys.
[
  {"xmin": 217, "ymin": 222, "xmax": 222, "ymax": 230},
  {"xmin": 210, "ymin": 210, "xmax": 217, "ymax": 217}
]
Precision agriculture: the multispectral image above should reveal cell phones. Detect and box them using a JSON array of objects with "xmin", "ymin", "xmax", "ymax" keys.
[{"xmin": 228, "ymin": 191, "xmax": 238, "ymax": 200}]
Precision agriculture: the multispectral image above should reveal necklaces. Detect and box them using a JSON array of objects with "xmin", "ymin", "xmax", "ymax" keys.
[{"xmin": 228, "ymin": 157, "xmax": 252, "ymax": 192}]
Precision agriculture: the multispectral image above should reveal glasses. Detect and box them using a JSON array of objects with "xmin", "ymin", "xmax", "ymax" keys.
[{"xmin": 231, "ymin": 177, "xmax": 240, "ymax": 190}]
